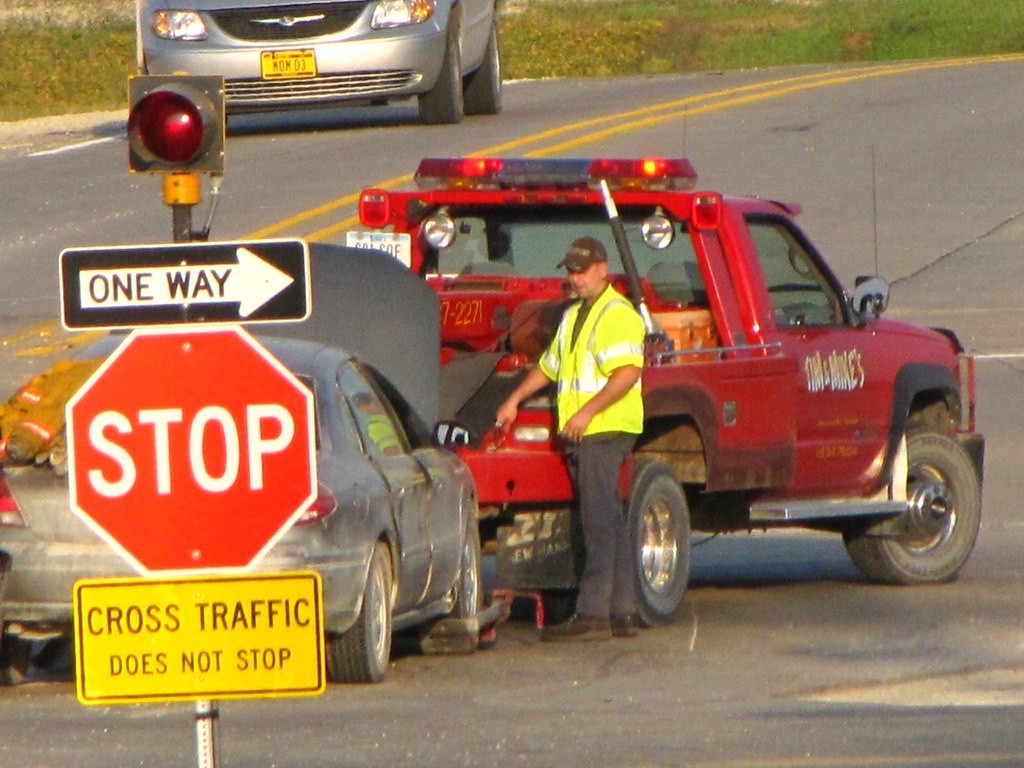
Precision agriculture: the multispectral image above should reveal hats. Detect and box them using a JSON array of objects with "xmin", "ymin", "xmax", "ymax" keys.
[{"xmin": 556, "ymin": 236, "xmax": 607, "ymax": 272}]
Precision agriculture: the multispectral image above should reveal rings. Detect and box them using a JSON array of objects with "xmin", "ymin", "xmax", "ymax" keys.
[{"xmin": 495, "ymin": 422, "xmax": 503, "ymax": 428}]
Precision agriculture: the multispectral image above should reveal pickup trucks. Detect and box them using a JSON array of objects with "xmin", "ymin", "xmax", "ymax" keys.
[{"xmin": 352, "ymin": 154, "xmax": 989, "ymax": 634}]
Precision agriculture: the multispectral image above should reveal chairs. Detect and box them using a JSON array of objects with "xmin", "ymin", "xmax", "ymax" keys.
[
  {"xmin": 452, "ymin": 261, "xmax": 521, "ymax": 294},
  {"xmin": 648, "ymin": 259, "xmax": 708, "ymax": 309}
]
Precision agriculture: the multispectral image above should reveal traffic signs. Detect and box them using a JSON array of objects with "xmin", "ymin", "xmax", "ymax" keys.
[{"xmin": 59, "ymin": 237, "xmax": 314, "ymax": 333}]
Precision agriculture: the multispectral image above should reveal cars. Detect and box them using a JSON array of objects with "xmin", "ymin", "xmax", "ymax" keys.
[
  {"xmin": 0, "ymin": 327, "xmax": 485, "ymax": 686},
  {"xmin": 133, "ymin": 0, "xmax": 503, "ymax": 126}
]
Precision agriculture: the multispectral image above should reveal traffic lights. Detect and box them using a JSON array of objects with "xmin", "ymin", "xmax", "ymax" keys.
[{"xmin": 119, "ymin": 70, "xmax": 226, "ymax": 175}]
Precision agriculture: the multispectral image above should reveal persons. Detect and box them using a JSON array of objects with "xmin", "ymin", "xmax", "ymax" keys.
[{"xmin": 494, "ymin": 234, "xmax": 643, "ymax": 644}]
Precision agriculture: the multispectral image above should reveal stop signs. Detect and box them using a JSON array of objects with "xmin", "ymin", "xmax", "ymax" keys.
[{"xmin": 66, "ymin": 325, "xmax": 317, "ymax": 578}]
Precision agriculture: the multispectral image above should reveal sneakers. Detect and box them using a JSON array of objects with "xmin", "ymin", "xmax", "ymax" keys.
[
  {"xmin": 611, "ymin": 612, "xmax": 636, "ymax": 636},
  {"xmin": 541, "ymin": 611, "xmax": 613, "ymax": 642}
]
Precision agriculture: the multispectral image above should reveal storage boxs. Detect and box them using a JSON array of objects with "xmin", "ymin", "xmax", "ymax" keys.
[{"xmin": 655, "ymin": 313, "xmax": 722, "ymax": 367}]
